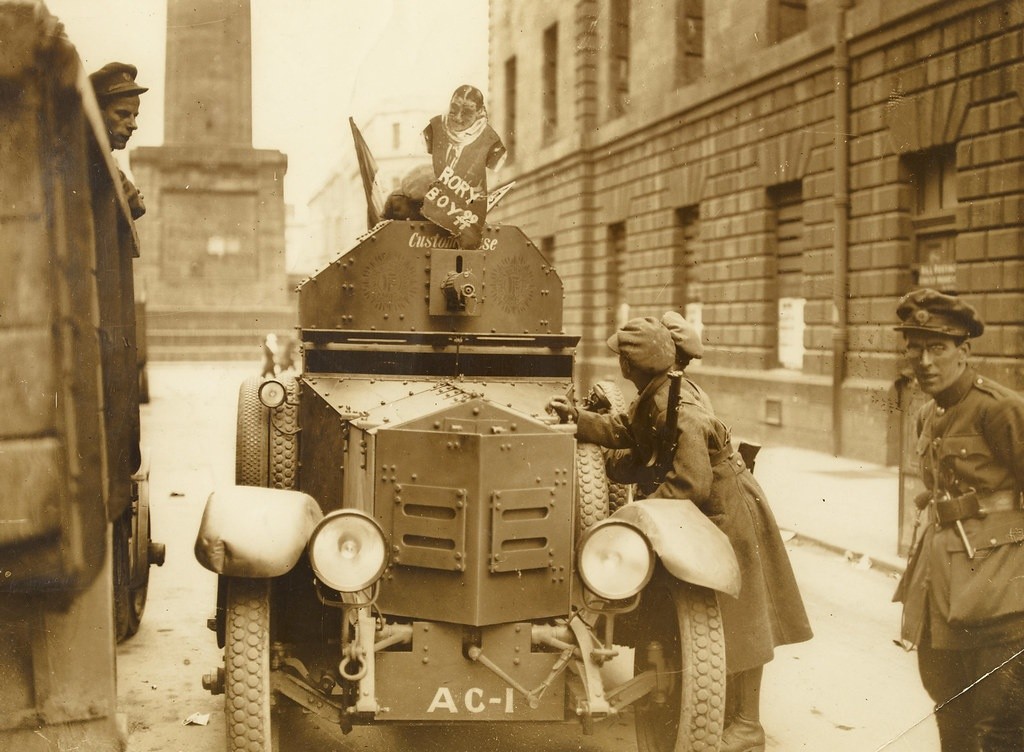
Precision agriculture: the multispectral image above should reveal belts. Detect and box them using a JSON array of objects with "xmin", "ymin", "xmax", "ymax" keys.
[
  {"xmin": 927, "ymin": 490, "xmax": 1024, "ymax": 520},
  {"xmin": 710, "ymin": 442, "xmax": 733, "ymax": 467}
]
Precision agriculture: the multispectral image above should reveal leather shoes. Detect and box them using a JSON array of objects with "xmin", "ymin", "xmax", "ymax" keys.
[{"xmin": 720, "ymin": 713, "xmax": 765, "ymax": 752}]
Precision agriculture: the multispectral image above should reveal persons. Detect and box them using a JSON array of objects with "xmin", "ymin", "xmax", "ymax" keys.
[
  {"xmin": 90, "ymin": 62, "xmax": 148, "ymax": 220},
  {"xmin": 892, "ymin": 290, "xmax": 1024, "ymax": 752},
  {"xmin": 546, "ymin": 310, "xmax": 814, "ymax": 752}
]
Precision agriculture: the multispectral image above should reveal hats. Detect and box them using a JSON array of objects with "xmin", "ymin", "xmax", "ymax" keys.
[
  {"xmin": 88, "ymin": 62, "xmax": 148, "ymax": 96},
  {"xmin": 660, "ymin": 311, "xmax": 704, "ymax": 360},
  {"xmin": 893, "ymin": 288, "xmax": 984, "ymax": 338},
  {"xmin": 607, "ymin": 316, "xmax": 676, "ymax": 375},
  {"xmin": 403, "ymin": 164, "xmax": 436, "ymax": 202}
]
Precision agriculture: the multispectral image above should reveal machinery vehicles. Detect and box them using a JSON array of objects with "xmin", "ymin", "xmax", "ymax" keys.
[{"xmin": 221, "ymin": 133, "xmax": 752, "ymax": 752}]
